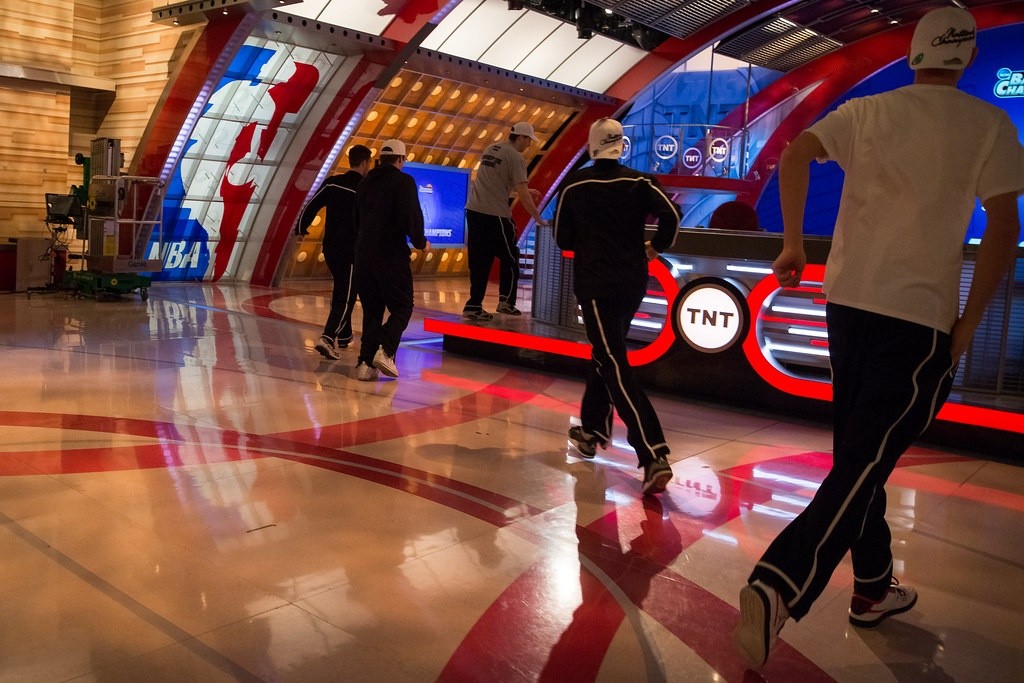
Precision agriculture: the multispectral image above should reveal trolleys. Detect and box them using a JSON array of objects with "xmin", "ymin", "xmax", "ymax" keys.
[{"xmin": 66, "ymin": 173, "xmax": 166, "ymax": 304}]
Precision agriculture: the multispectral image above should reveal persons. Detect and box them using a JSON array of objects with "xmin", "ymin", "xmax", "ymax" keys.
[
  {"xmin": 737, "ymin": 10, "xmax": 1023, "ymax": 670},
  {"xmin": 352, "ymin": 139, "xmax": 431, "ymax": 380},
  {"xmin": 553, "ymin": 119, "xmax": 673, "ymax": 495},
  {"xmin": 464, "ymin": 122, "xmax": 551, "ymax": 320},
  {"xmin": 295, "ymin": 145, "xmax": 372, "ymax": 360}
]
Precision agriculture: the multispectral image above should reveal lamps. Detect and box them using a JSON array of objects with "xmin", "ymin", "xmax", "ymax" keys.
[
  {"xmin": 574, "ymin": 0, "xmax": 593, "ymax": 39},
  {"xmin": 631, "ymin": 22, "xmax": 654, "ymax": 50}
]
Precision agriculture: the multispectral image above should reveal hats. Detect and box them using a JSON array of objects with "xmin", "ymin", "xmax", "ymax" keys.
[
  {"xmin": 380, "ymin": 139, "xmax": 407, "ymax": 156},
  {"xmin": 510, "ymin": 122, "xmax": 539, "ymax": 141},
  {"xmin": 909, "ymin": 6, "xmax": 976, "ymax": 71},
  {"xmin": 588, "ymin": 117, "xmax": 623, "ymax": 159}
]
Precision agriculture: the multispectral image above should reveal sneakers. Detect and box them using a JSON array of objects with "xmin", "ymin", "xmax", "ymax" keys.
[
  {"xmin": 847, "ymin": 575, "xmax": 918, "ymax": 627},
  {"xmin": 640, "ymin": 454, "xmax": 673, "ymax": 495},
  {"xmin": 372, "ymin": 345, "xmax": 400, "ymax": 377},
  {"xmin": 338, "ymin": 336, "xmax": 355, "ymax": 347},
  {"xmin": 314, "ymin": 337, "xmax": 341, "ymax": 360},
  {"xmin": 496, "ymin": 301, "xmax": 521, "ymax": 315},
  {"xmin": 462, "ymin": 304, "xmax": 493, "ymax": 320},
  {"xmin": 567, "ymin": 424, "xmax": 596, "ymax": 458},
  {"xmin": 356, "ymin": 361, "xmax": 379, "ymax": 380},
  {"xmin": 737, "ymin": 579, "xmax": 789, "ymax": 671}
]
